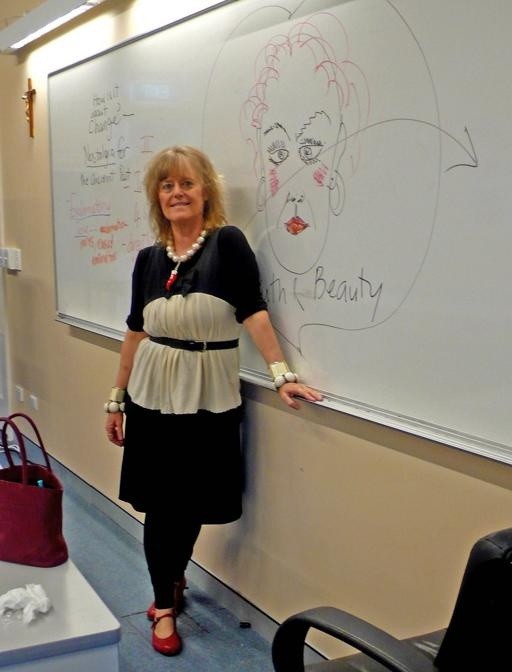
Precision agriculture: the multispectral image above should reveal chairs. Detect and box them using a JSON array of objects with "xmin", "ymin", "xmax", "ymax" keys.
[{"xmin": 268, "ymin": 523, "xmax": 511, "ymax": 671}]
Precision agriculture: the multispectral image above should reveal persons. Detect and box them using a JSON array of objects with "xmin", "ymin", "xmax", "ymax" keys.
[
  {"xmin": 106, "ymin": 145, "xmax": 323, "ymax": 654},
  {"xmin": 239, "ymin": 12, "xmax": 370, "ymax": 275}
]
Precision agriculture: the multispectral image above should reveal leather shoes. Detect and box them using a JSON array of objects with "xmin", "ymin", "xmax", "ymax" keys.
[{"xmin": 146, "ymin": 579, "xmax": 186, "ymax": 656}]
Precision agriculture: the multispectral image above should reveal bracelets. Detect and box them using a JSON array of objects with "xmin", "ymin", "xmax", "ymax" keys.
[
  {"xmin": 269, "ymin": 360, "xmax": 300, "ymax": 391},
  {"xmin": 103, "ymin": 387, "xmax": 126, "ymax": 413}
]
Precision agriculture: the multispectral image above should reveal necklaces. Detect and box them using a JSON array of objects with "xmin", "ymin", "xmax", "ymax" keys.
[{"xmin": 166, "ymin": 226, "xmax": 207, "ymax": 291}]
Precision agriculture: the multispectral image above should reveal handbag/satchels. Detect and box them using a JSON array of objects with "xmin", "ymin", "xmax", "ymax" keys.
[{"xmin": 0, "ymin": 413, "xmax": 69, "ymax": 568}]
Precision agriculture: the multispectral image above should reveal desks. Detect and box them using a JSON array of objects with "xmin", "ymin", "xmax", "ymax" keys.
[{"xmin": 0, "ymin": 555, "xmax": 122, "ymax": 671}]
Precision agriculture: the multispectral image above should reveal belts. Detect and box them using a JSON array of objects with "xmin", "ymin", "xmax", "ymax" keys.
[{"xmin": 149, "ymin": 335, "xmax": 239, "ymax": 351}]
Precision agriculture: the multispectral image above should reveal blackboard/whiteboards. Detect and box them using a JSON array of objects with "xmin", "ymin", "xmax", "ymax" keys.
[{"xmin": 47, "ymin": 1, "xmax": 510, "ymax": 468}]
